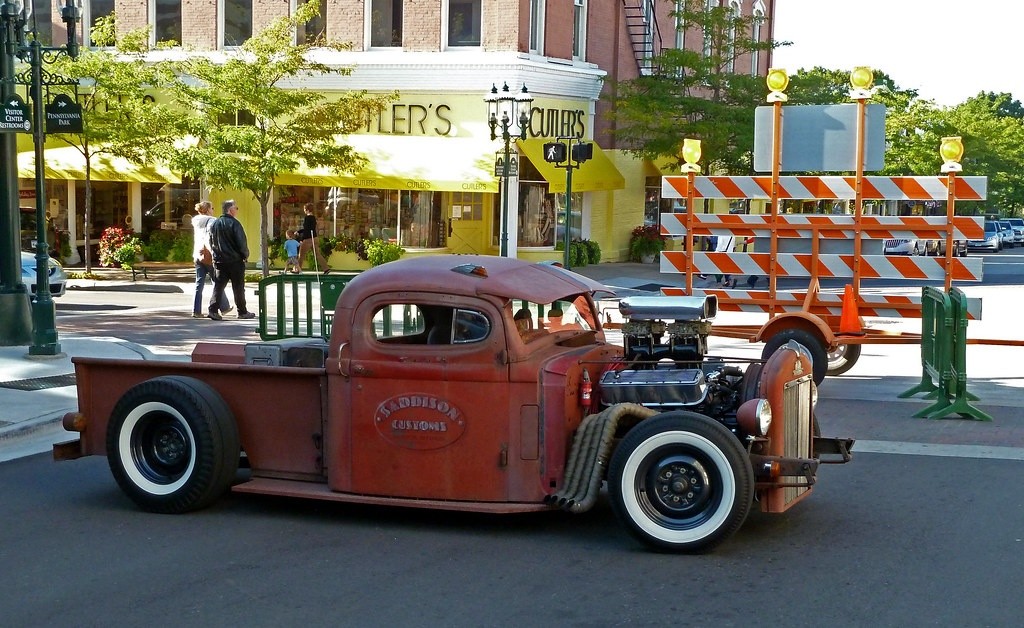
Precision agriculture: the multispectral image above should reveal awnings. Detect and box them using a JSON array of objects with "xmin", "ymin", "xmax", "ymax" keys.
[
  {"xmin": 520, "ymin": 142, "xmax": 626, "ymax": 194},
  {"xmin": 18, "ymin": 134, "xmax": 504, "ymax": 194}
]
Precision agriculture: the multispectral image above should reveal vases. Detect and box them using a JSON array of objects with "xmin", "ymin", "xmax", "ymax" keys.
[{"xmin": 639, "ymin": 254, "xmax": 655, "ymax": 264}]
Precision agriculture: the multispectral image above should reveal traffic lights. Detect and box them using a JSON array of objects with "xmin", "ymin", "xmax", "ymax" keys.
[
  {"xmin": 571, "ymin": 142, "xmax": 593, "ymax": 162},
  {"xmin": 543, "ymin": 142, "xmax": 566, "ymax": 162}
]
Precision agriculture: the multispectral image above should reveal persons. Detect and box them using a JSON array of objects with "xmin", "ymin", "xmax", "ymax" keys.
[
  {"xmin": 290, "ymin": 203, "xmax": 331, "ymax": 275},
  {"xmin": 279, "ymin": 229, "xmax": 303, "ymax": 275},
  {"xmin": 207, "ymin": 201, "xmax": 255, "ymax": 320},
  {"xmin": 191, "ymin": 200, "xmax": 233, "ymax": 318},
  {"xmin": 681, "ymin": 236, "xmax": 770, "ymax": 287},
  {"xmin": 45, "ymin": 211, "xmax": 56, "ymax": 251}
]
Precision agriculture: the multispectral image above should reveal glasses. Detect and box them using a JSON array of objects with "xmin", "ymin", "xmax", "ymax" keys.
[
  {"xmin": 208, "ymin": 208, "xmax": 213, "ymax": 212},
  {"xmin": 232, "ymin": 207, "xmax": 238, "ymax": 211}
]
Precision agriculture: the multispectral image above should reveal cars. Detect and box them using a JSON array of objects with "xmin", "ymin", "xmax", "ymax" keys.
[
  {"xmin": 20, "ymin": 250, "xmax": 67, "ymax": 304},
  {"xmin": 882, "ymin": 216, "xmax": 1024, "ymax": 258}
]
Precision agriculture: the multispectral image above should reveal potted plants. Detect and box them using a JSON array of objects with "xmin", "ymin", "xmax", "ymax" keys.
[{"xmin": 112, "ymin": 238, "xmax": 143, "ymax": 270}]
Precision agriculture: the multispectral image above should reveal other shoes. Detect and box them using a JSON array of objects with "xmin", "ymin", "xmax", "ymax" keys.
[
  {"xmin": 237, "ymin": 311, "xmax": 255, "ymax": 319},
  {"xmin": 192, "ymin": 312, "xmax": 207, "ymax": 318},
  {"xmin": 279, "ymin": 270, "xmax": 284, "ymax": 274},
  {"xmin": 221, "ymin": 306, "xmax": 233, "ymax": 316},
  {"xmin": 291, "ymin": 270, "xmax": 299, "ymax": 274},
  {"xmin": 207, "ymin": 312, "xmax": 222, "ymax": 321},
  {"xmin": 323, "ymin": 269, "xmax": 331, "ymax": 275}
]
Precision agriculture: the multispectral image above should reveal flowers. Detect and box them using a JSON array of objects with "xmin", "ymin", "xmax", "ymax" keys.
[
  {"xmin": 96, "ymin": 227, "xmax": 134, "ymax": 268},
  {"xmin": 630, "ymin": 224, "xmax": 666, "ymax": 261}
]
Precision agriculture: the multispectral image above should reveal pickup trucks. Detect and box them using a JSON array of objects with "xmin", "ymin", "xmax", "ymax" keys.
[{"xmin": 47, "ymin": 250, "xmax": 856, "ymax": 560}]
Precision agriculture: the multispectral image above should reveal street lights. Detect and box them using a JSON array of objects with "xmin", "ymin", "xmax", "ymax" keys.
[
  {"xmin": 483, "ymin": 81, "xmax": 535, "ymax": 257},
  {"xmin": 0, "ymin": 0, "xmax": 84, "ymax": 356}
]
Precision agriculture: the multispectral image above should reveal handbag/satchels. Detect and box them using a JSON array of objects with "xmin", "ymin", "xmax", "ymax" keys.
[{"xmin": 199, "ymin": 245, "xmax": 213, "ymax": 266}]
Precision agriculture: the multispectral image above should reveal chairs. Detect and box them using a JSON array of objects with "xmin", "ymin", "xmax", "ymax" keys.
[{"xmin": 426, "ymin": 325, "xmax": 449, "ymax": 342}]
